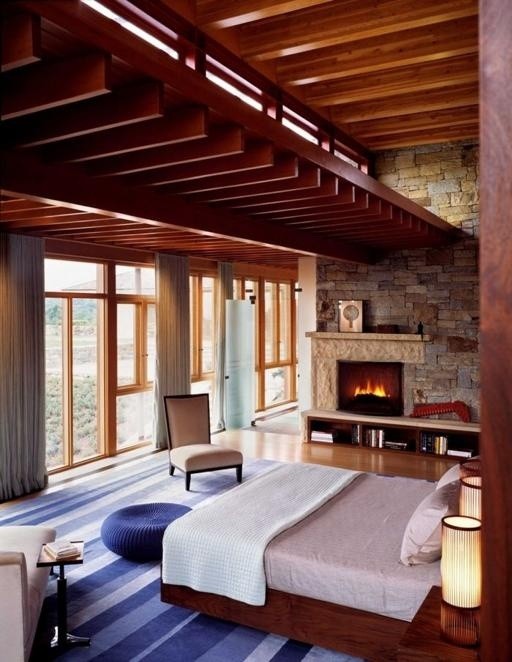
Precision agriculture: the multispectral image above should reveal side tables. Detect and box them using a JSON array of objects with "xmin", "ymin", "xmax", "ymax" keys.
[{"xmin": 35, "ymin": 541, "xmax": 90, "ymax": 662}]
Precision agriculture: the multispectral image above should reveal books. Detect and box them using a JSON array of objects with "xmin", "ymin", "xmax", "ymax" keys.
[
  {"xmin": 44, "ymin": 538, "xmax": 81, "ymax": 560},
  {"xmin": 309, "ymin": 424, "xmax": 474, "ymax": 458}
]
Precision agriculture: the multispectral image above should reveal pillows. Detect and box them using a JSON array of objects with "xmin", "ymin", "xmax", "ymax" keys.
[
  {"xmin": 400, "ymin": 477, "xmax": 463, "ymax": 566},
  {"xmin": 436, "ymin": 454, "xmax": 480, "ymax": 488}
]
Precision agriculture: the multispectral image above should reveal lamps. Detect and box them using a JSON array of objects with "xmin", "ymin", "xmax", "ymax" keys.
[
  {"xmin": 460, "ymin": 459, "xmax": 481, "ymax": 477},
  {"xmin": 460, "ymin": 476, "xmax": 482, "ymax": 520},
  {"xmin": 440, "ymin": 515, "xmax": 482, "ymax": 647}
]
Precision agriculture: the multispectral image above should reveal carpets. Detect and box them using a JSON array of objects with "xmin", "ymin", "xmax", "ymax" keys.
[{"xmin": 0, "ymin": 455, "xmax": 441, "ymax": 662}]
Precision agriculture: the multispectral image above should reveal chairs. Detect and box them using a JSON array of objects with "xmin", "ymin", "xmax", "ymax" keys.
[
  {"xmin": 0, "ymin": 525, "xmax": 58, "ymax": 662},
  {"xmin": 162, "ymin": 394, "xmax": 243, "ymax": 490}
]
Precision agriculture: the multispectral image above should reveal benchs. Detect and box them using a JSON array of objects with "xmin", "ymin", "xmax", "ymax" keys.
[{"xmin": 302, "ymin": 410, "xmax": 481, "ymax": 443}]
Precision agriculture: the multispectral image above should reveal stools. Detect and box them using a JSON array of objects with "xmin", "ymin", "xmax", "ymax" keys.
[{"xmin": 101, "ymin": 503, "xmax": 193, "ymax": 561}]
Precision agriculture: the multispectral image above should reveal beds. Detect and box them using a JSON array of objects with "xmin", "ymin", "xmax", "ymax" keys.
[{"xmin": 159, "ymin": 461, "xmax": 441, "ymax": 662}]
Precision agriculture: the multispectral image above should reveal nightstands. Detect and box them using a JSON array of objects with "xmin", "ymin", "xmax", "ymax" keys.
[{"xmin": 398, "ymin": 585, "xmax": 479, "ymax": 662}]
